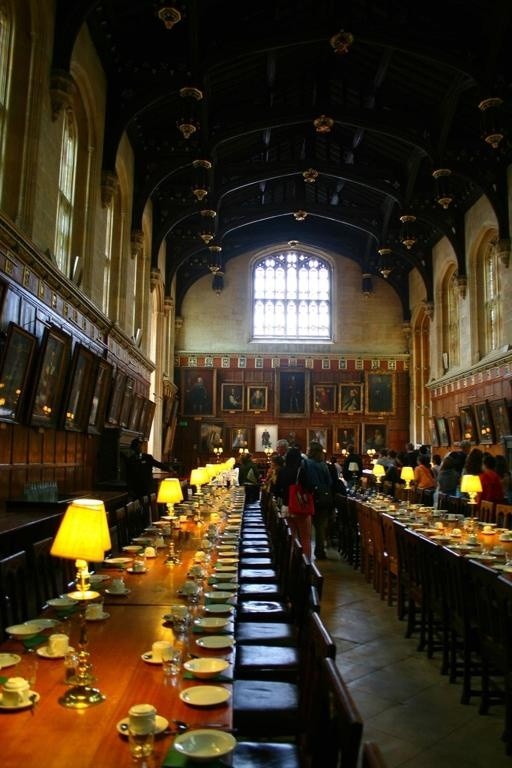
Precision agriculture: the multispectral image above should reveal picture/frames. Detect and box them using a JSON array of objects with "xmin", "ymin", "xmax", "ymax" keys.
[
  {"xmin": 311, "ymin": 382, "xmax": 337, "ymax": 414},
  {"xmin": 427, "ymin": 398, "xmax": 512, "ymax": 447},
  {"xmin": 220, "ymin": 383, "xmax": 246, "ymax": 412},
  {"xmin": 245, "ymin": 385, "xmax": 268, "ymax": 412},
  {"xmin": 362, "ymin": 370, "xmax": 396, "ymax": 416},
  {"xmin": 174, "ymin": 354, "xmax": 409, "ymax": 371},
  {"xmin": 177, "ymin": 366, "xmax": 218, "ymax": 418},
  {"xmin": 197, "ymin": 420, "xmax": 389, "ymax": 460},
  {"xmin": 273, "ymin": 366, "xmax": 312, "ymax": 419},
  {"xmin": 336, "ymin": 383, "xmax": 364, "ymax": 414},
  {"xmin": 1, "ymin": 320, "xmax": 156, "ymax": 440}
]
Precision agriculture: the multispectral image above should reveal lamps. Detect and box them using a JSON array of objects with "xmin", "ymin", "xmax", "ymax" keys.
[
  {"xmin": 198, "ymin": 205, "xmax": 217, "ymax": 245},
  {"xmin": 358, "ymin": 90, "xmax": 504, "ymax": 302},
  {"xmin": 286, "ymin": 27, "xmax": 356, "ymax": 248},
  {"xmin": 204, "ymin": 239, "xmax": 223, "ymax": 273},
  {"xmin": 177, "ymin": 85, "xmax": 205, "ymax": 140},
  {"xmin": 151, "ymin": 0, "xmax": 188, "ymax": 29},
  {"xmin": 47, "ymin": 502, "xmax": 107, "ymax": 710},
  {"xmin": 189, "ymin": 155, "xmax": 213, "ymax": 201},
  {"xmin": 61, "ymin": 497, "xmax": 113, "ymax": 686},
  {"xmin": 211, "ymin": 269, "xmax": 224, "ymax": 296}
]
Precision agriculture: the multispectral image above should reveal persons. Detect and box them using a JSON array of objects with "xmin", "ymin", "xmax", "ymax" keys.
[
  {"xmin": 128, "ymin": 439, "xmax": 174, "ymax": 535},
  {"xmin": 232, "ymin": 439, "xmax": 510, "ymax": 559}
]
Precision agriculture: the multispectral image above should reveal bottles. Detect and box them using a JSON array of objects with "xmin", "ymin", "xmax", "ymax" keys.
[{"xmin": 64, "ymin": 645, "xmax": 93, "ymax": 686}]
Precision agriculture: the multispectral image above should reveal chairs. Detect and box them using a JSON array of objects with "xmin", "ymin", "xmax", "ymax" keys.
[
  {"xmin": 360, "ymin": 740, "xmax": 388, "ymax": 767},
  {"xmin": 231, "ymin": 611, "xmax": 337, "ymax": 741},
  {"xmin": 229, "ymin": 655, "xmax": 363, "ymax": 766},
  {"xmin": 235, "ymin": 584, "xmax": 320, "ymax": 681}
]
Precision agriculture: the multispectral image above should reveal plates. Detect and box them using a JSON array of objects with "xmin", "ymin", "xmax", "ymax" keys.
[
  {"xmin": 142, "ymin": 651, "xmax": 165, "ymax": 663},
  {"xmin": 1, "ymin": 690, "xmax": 40, "ymax": 710},
  {"xmin": 116, "ymin": 715, "xmax": 169, "ymax": 735},
  {"xmin": 105, "ymin": 587, "xmax": 131, "ymax": 596},
  {"xmin": 85, "ymin": 613, "xmax": 110, "ymax": 620},
  {"xmin": 37, "ymin": 645, "xmax": 74, "ymax": 658},
  {"xmin": 365, "ymin": 500, "xmax": 511, "ymax": 572},
  {"xmin": 0, "ymin": 652, "xmax": 21, "ymax": 667}
]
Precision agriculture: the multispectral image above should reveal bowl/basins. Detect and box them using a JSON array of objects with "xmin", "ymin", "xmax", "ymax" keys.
[
  {"xmin": 179, "ymin": 686, "xmax": 232, "ymax": 707},
  {"xmin": 6, "ymin": 624, "xmax": 45, "ymax": 638},
  {"xmin": 194, "ymin": 617, "xmax": 230, "ymax": 632},
  {"xmin": 184, "ymin": 657, "xmax": 230, "ymax": 676},
  {"xmin": 103, "ymin": 519, "xmax": 180, "ymax": 575},
  {"xmin": 177, "ymin": 729, "xmax": 237, "ymax": 761},
  {"xmin": 88, "ymin": 574, "xmax": 112, "ymax": 584},
  {"xmin": 201, "ymin": 483, "xmax": 246, "ymax": 614},
  {"xmin": 47, "ymin": 598, "xmax": 78, "ymax": 610},
  {"xmin": 196, "ymin": 635, "xmax": 233, "ymax": 649},
  {"xmin": 25, "ymin": 620, "xmax": 61, "ymax": 628}
]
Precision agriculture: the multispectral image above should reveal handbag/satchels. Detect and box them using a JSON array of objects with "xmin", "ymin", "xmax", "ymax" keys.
[
  {"xmin": 288, "ymin": 484, "xmax": 314, "ymax": 514},
  {"xmin": 246, "ymin": 468, "xmax": 257, "ymax": 483}
]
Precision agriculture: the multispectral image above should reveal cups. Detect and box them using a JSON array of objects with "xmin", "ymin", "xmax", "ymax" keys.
[
  {"xmin": 128, "ymin": 717, "xmax": 155, "ymax": 759},
  {"xmin": 48, "ymin": 634, "xmax": 69, "ymax": 655},
  {"xmin": 86, "ymin": 604, "xmax": 103, "ymax": 617},
  {"xmin": 370, "ymin": 494, "xmax": 511, "ymax": 555},
  {"xmin": 163, "ymin": 649, "xmax": 181, "ymax": 675},
  {"xmin": 128, "ymin": 703, "xmax": 156, "ymax": 733},
  {"xmin": 151, "ymin": 640, "xmax": 173, "ymax": 661},
  {"xmin": 2, "ymin": 676, "xmax": 30, "ymax": 706},
  {"xmin": 113, "ymin": 579, "xmax": 125, "ymax": 591},
  {"xmin": 161, "ymin": 486, "xmax": 229, "ymax": 623}
]
